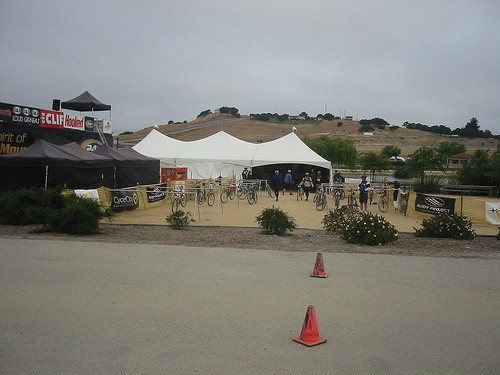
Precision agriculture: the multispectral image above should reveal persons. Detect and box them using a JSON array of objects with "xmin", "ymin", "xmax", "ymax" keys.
[
  {"xmin": 242, "ymin": 168, "xmax": 252, "ymax": 179},
  {"xmin": 299, "ymin": 173, "xmax": 314, "ymax": 201},
  {"xmin": 283, "ymin": 170, "xmax": 294, "ymax": 195},
  {"xmin": 358, "ymin": 176, "xmax": 370, "ymax": 214},
  {"xmin": 334, "ymin": 170, "xmax": 345, "ymax": 194},
  {"xmin": 315, "ymin": 171, "xmax": 323, "ymax": 193},
  {"xmin": 272, "ymin": 170, "xmax": 282, "ymax": 201}
]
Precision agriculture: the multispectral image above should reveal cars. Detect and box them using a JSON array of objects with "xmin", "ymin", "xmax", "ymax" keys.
[{"xmin": 389, "ymin": 157, "xmax": 405, "ymax": 162}]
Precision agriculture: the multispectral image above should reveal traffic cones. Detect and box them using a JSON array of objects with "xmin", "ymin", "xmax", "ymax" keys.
[
  {"xmin": 291, "ymin": 304, "xmax": 327, "ymax": 347},
  {"xmin": 309, "ymin": 252, "xmax": 328, "ymax": 278}
]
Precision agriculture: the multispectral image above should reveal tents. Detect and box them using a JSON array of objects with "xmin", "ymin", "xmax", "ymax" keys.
[
  {"xmin": 0, "ymin": 138, "xmax": 160, "ymax": 211},
  {"xmin": 61, "ymin": 90, "xmax": 112, "ymax": 133},
  {"xmin": 132, "ymin": 129, "xmax": 333, "ymax": 221}
]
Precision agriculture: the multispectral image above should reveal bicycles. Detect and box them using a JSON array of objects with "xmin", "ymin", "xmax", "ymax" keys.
[
  {"xmin": 346, "ymin": 188, "xmax": 358, "ymax": 210},
  {"xmin": 313, "ymin": 186, "xmax": 327, "ymax": 211},
  {"xmin": 220, "ymin": 179, "xmax": 260, "ymax": 205},
  {"xmin": 171, "ymin": 185, "xmax": 187, "ymax": 215},
  {"xmin": 296, "ymin": 184, "xmax": 305, "ymax": 201},
  {"xmin": 330, "ymin": 182, "xmax": 346, "ymax": 209},
  {"xmin": 376, "ymin": 189, "xmax": 389, "ymax": 213},
  {"xmin": 265, "ymin": 184, "xmax": 274, "ymax": 198},
  {"xmin": 194, "ymin": 179, "xmax": 216, "ymax": 206}
]
